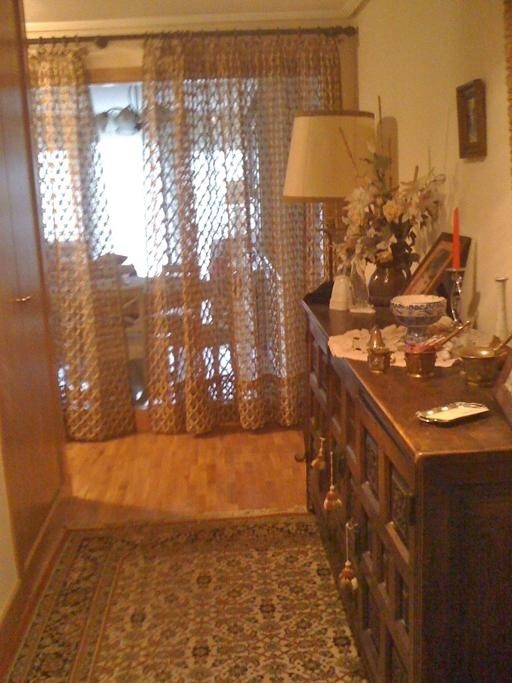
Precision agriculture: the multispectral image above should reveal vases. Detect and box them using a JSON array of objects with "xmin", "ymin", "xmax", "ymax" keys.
[{"xmin": 367, "ymin": 264, "xmax": 403, "ymax": 305}]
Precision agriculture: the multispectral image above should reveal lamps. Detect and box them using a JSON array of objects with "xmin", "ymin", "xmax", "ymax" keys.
[
  {"xmin": 282, "ymin": 111, "xmax": 375, "ymax": 303},
  {"xmin": 93, "ymin": 83, "xmax": 195, "ymax": 137}
]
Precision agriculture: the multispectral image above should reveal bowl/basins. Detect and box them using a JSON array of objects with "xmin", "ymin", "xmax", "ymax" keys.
[{"xmin": 451, "ymin": 347, "xmax": 509, "ymax": 387}]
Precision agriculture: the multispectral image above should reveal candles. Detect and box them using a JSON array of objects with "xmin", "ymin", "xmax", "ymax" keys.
[{"xmin": 452, "ymin": 206, "xmax": 461, "ymax": 269}]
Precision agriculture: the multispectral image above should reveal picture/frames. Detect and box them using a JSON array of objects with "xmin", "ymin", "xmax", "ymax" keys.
[
  {"xmin": 491, "ymin": 352, "xmax": 512, "ymax": 426},
  {"xmin": 456, "ymin": 78, "xmax": 487, "ymax": 159},
  {"xmin": 401, "ymin": 232, "xmax": 471, "ymax": 295}
]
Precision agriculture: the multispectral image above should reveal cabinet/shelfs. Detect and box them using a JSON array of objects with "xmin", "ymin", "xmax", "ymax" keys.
[{"xmin": 0, "ymin": 0, "xmax": 73, "ymax": 583}]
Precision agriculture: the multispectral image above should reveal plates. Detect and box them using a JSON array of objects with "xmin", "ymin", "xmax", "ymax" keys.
[{"xmin": 414, "ymin": 401, "xmax": 490, "ymax": 424}]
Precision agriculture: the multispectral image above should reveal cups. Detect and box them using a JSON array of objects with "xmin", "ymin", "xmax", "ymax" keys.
[
  {"xmin": 405, "ymin": 351, "xmax": 439, "ymax": 379},
  {"xmin": 368, "ymin": 347, "xmax": 391, "ymax": 374}
]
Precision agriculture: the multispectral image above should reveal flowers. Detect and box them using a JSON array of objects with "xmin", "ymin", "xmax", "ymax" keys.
[{"xmin": 334, "ymin": 165, "xmax": 444, "ymax": 287}]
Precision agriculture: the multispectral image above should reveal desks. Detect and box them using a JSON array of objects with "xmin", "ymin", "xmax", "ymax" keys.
[{"xmin": 299, "ymin": 298, "xmax": 512, "ymax": 683}]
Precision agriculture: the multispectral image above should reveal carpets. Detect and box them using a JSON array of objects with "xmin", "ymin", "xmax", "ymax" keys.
[{"xmin": 0, "ymin": 512, "xmax": 367, "ymax": 683}]
